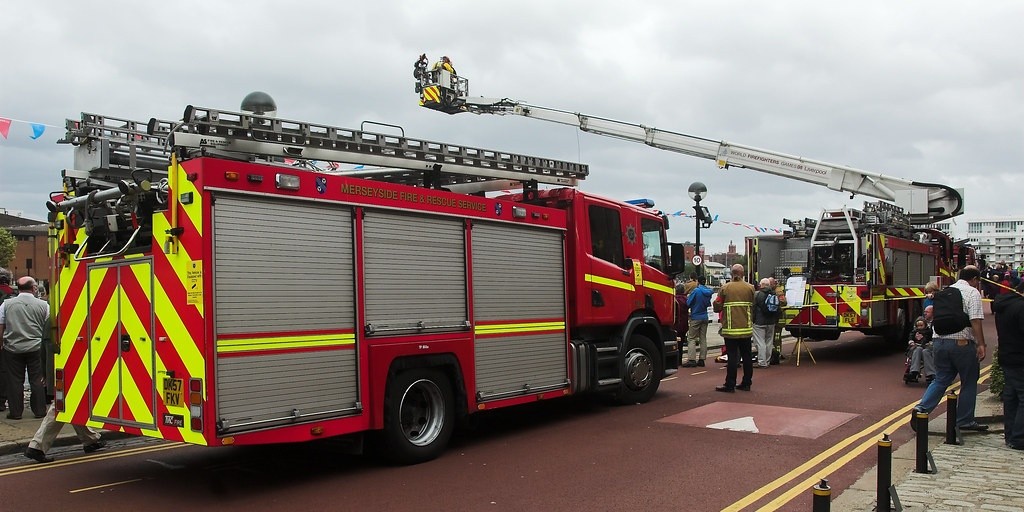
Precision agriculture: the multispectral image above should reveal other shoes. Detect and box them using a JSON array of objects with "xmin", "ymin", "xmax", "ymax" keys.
[
  {"xmin": 904, "ymin": 372, "xmax": 918, "ymax": 380},
  {"xmin": 696, "ymin": 360, "xmax": 704, "ymax": 366},
  {"xmin": 0, "ymin": 404, "xmax": 6, "ymax": 412},
  {"xmin": 736, "ymin": 383, "xmax": 750, "ymax": 391},
  {"xmin": 753, "ymin": 363, "xmax": 767, "ymax": 369},
  {"xmin": 7, "ymin": 412, "xmax": 23, "ymax": 420},
  {"xmin": 715, "ymin": 384, "xmax": 735, "ymax": 393},
  {"xmin": 926, "ymin": 374, "xmax": 935, "ymax": 380},
  {"xmin": 683, "ymin": 359, "xmax": 697, "ymax": 367},
  {"xmin": 36, "ymin": 413, "xmax": 46, "ymax": 418}
]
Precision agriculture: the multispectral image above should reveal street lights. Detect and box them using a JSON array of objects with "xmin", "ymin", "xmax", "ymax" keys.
[{"xmin": 687, "ymin": 180, "xmax": 712, "ymax": 286}]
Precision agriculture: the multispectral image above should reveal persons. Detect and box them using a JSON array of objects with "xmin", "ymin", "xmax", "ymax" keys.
[
  {"xmin": 909, "ymin": 264, "xmax": 988, "ymax": 433},
  {"xmin": 0, "ymin": 267, "xmax": 53, "ymax": 419},
  {"xmin": 433, "ymin": 56, "xmax": 456, "ymax": 101},
  {"xmin": 978, "ymin": 256, "xmax": 1024, "ymax": 314},
  {"xmin": 994, "ymin": 280, "xmax": 1024, "ymax": 449},
  {"xmin": 675, "ymin": 272, "xmax": 714, "ymax": 366},
  {"xmin": 713, "ymin": 263, "xmax": 755, "ymax": 393},
  {"xmin": 908, "ymin": 282, "xmax": 942, "ymax": 382},
  {"xmin": 753, "ymin": 277, "xmax": 787, "ymax": 368}
]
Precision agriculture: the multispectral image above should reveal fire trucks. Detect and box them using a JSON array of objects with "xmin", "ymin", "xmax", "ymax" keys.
[
  {"xmin": 44, "ymin": 104, "xmax": 688, "ymax": 464},
  {"xmin": 414, "ymin": 68, "xmax": 987, "ymax": 349}
]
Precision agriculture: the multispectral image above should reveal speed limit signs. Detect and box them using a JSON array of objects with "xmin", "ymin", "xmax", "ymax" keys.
[{"xmin": 690, "ymin": 255, "xmax": 702, "ymax": 266}]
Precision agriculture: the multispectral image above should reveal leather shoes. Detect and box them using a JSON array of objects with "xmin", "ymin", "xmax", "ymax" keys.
[
  {"xmin": 960, "ymin": 423, "xmax": 989, "ymax": 430},
  {"xmin": 910, "ymin": 407, "xmax": 923, "ymax": 431}
]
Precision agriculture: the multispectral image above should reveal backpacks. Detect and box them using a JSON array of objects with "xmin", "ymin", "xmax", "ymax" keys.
[
  {"xmin": 760, "ymin": 290, "xmax": 779, "ymax": 315},
  {"xmin": 932, "ymin": 285, "xmax": 969, "ymax": 335}
]
